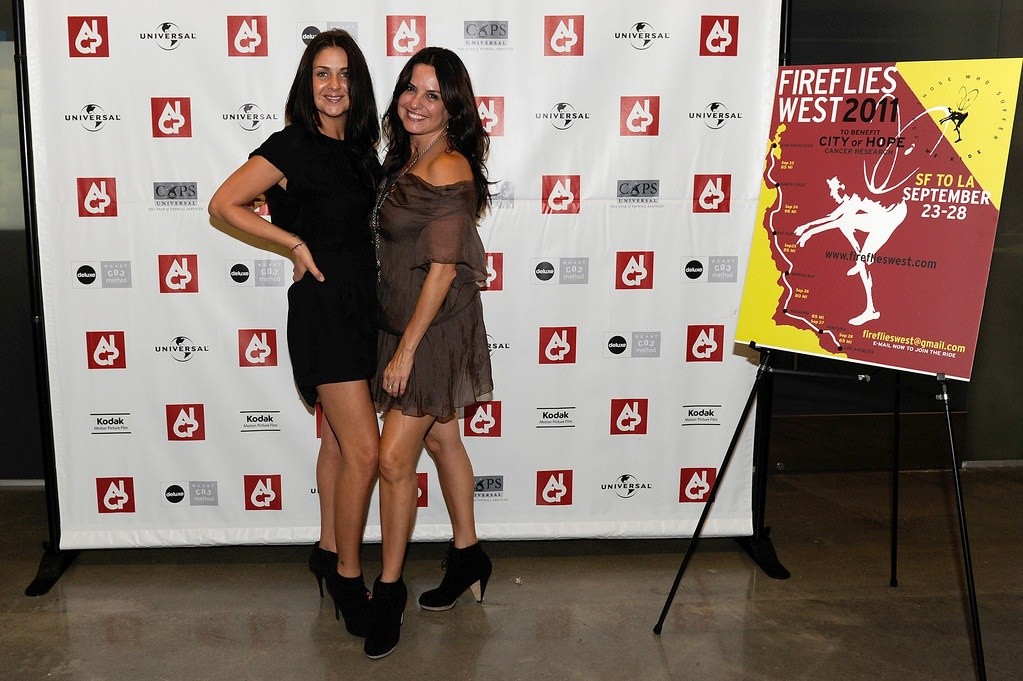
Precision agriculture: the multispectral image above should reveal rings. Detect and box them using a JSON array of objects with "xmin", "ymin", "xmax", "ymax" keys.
[{"xmin": 387, "ymin": 385, "xmax": 393, "ymax": 389}]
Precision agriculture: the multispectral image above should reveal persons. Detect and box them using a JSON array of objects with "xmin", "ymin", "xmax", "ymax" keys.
[
  {"xmin": 364, "ymin": 48, "xmax": 494, "ymax": 657},
  {"xmin": 206, "ymin": 31, "xmax": 383, "ymax": 637}
]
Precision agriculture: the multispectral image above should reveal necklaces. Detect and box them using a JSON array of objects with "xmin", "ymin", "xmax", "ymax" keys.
[{"xmin": 373, "ymin": 132, "xmax": 447, "ymax": 282}]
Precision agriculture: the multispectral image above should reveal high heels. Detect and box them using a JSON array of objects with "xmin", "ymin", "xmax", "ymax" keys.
[
  {"xmin": 326, "ymin": 570, "xmax": 376, "ymax": 638},
  {"xmin": 417, "ymin": 538, "xmax": 492, "ymax": 611},
  {"xmin": 364, "ymin": 572, "xmax": 407, "ymax": 660},
  {"xmin": 309, "ymin": 541, "xmax": 373, "ymax": 602}
]
